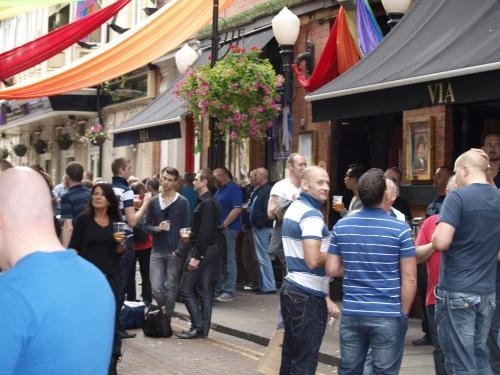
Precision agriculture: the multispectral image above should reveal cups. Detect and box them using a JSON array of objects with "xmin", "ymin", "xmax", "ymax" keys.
[
  {"xmin": 333, "ymin": 196, "xmax": 343, "ymax": 211},
  {"xmin": 180, "ymin": 228, "xmax": 190, "ymax": 244},
  {"xmin": 163, "ymin": 223, "xmax": 170, "ymax": 231},
  {"xmin": 134, "ymin": 195, "xmax": 139, "ymax": 208},
  {"xmin": 328, "ymin": 316, "xmax": 336, "ymax": 326},
  {"xmin": 113, "ymin": 222, "xmax": 126, "ymax": 241}
]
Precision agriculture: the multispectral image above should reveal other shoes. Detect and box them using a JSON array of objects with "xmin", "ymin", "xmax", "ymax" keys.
[
  {"xmin": 120, "ymin": 331, "xmax": 136, "ymax": 338},
  {"xmin": 254, "ymin": 288, "xmax": 277, "ymax": 294},
  {"xmin": 215, "ymin": 292, "xmax": 234, "ymax": 302}
]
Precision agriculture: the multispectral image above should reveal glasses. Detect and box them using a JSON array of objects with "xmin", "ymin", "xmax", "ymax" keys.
[{"xmin": 345, "ymin": 173, "xmax": 355, "ymax": 179}]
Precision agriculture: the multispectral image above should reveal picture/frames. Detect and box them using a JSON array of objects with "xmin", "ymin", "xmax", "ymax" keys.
[{"xmin": 405, "ymin": 114, "xmax": 434, "ymax": 179}]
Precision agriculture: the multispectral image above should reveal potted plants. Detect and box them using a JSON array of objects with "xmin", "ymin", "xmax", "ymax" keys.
[
  {"xmin": 14, "ymin": 144, "xmax": 27, "ymax": 156},
  {"xmin": 34, "ymin": 139, "xmax": 49, "ymax": 154},
  {"xmin": 56, "ymin": 133, "xmax": 73, "ymax": 150}
]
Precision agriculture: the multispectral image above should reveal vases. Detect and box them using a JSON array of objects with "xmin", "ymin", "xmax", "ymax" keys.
[{"xmin": 90, "ymin": 137, "xmax": 106, "ymax": 146}]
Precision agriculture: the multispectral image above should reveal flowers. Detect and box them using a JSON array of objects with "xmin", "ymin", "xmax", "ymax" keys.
[
  {"xmin": 173, "ymin": 47, "xmax": 285, "ymax": 154},
  {"xmin": 79, "ymin": 123, "xmax": 112, "ymax": 145}
]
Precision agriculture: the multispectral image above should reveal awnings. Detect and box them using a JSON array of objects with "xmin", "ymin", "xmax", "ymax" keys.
[
  {"xmin": 305, "ymin": 1, "xmax": 499, "ymax": 122},
  {"xmin": 109, "ymin": 22, "xmax": 300, "ymax": 145}
]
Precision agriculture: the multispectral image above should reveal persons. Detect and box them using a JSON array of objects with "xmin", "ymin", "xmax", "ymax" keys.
[
  {"xmin": 414, "ymin": 175, "xmax": 454, "ymax": 375},
  {"xmin": 279, "ymin": 165, "xmax": 334, "ymax": 375},
  {"xmin": 480, "ymin": 130, "xmax": 500, "ymax": 184},
  {"xmin": 45, "ymin": 151, "xmax": 284, "ymax": 302},
  {"xmin": 176, "ymin": 168, "xmax": 219, "ymax": 339},
  {"xmin": 266, "ymin": 152, "xmax": 312, "ymax": 334},
  {"xmin": 430, "ymin": 148, "xmax": 500, "ymax": 375},
  {"xmin": 141, "ymin": 167, "xmax": 193, "ymax": 338},
  {"xmin": 335, "ymin": 160, "xmax": 455, "ymax": 346},
  {"xmin": 324, "ymin": 166, "xmax": 419, "ymax": 375},
  {"xmin": 0, "ymin": 167, "xmax": 118, "ymax": 375}
]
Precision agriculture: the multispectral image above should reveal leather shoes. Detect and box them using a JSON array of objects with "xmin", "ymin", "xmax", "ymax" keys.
[
  {"xmin": 175, "ymin": 328, "xmax": 206, "ymax": 339},
  {"xmin": 412, "ymin": 337, "xmax": 432, "ymax": 345}
]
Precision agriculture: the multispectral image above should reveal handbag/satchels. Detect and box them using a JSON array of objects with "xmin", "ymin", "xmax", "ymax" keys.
[
  {"xmin": 122, "ymin": 300, "xmax": 147, "ymax": 331},
  {"xmin": 142, "ymin": 302, "xmax": 173, "ymax": 338},
  {"xmin": 131, "ymin": 208, "xmax": 147, "ymax": 241}
]
